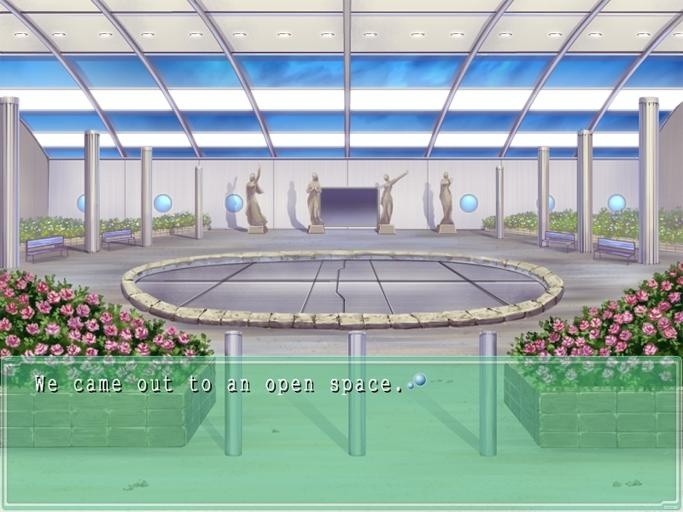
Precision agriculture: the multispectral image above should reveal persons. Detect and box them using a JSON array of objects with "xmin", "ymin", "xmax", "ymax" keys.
[
  {"xmin": 376, "ymin": 172, "xmax": 407, "ymax": 224},
  {"xmin": 306, "ymin": 172, "xmax": 325, "ymax": 224},
  {"xmin": 438, "ymin": 172, "xmax": 454, "ymax": 224},
  {"xmin": 244, "ymin": 166, "xmax": 269, "ymax": 227}
]
